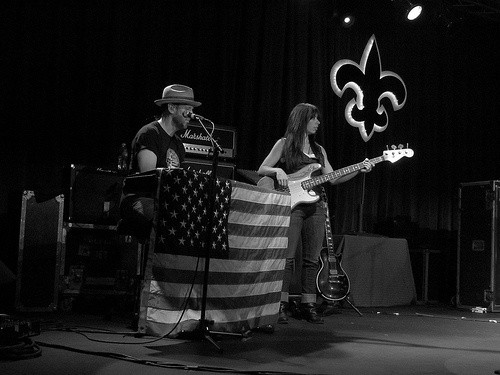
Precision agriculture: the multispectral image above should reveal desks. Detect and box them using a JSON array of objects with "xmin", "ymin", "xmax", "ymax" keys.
[{"xmin": 331, "ymin": 234, "xmax": 416, "ymax": 309}]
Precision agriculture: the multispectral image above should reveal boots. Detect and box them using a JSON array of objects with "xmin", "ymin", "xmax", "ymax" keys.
[
  {"xmin": 278, "ymin": 302, "xmax": 288, "ymax": 323},
  {"xmin": 305, "ymin": 303, "xmax": 324, "ymax": 323}
]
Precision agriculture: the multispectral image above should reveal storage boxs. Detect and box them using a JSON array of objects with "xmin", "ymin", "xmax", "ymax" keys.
[
  {"xmin": 456, "ymin": 180, "xmax": 500, "ymax": 314},
  {"xmin": 68, "ymin": 164, "xmax": 120, "ymax": 229},
  {"xmin": 17, "ymin": 189, "xmax": 65, "ymax": 312}
]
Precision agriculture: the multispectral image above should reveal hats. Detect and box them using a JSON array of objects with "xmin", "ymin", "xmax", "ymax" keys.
[{"xmin": 155, "ymin": 85, "xmax": 202, "ymax": 107}]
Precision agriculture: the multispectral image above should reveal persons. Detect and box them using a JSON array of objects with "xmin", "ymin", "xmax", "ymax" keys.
[
  {"xmin": 118, "ymin": 84, "xmax": 201, "ymax": 242},
  {"xmin": 258, "ymin": 103, "xmax": 375, "ymax": 324}
]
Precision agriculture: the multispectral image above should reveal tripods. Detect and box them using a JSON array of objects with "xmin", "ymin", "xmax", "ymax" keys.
[{"xmin": 168, "ymin": 117, "xmax": 244, "ymax": 355}]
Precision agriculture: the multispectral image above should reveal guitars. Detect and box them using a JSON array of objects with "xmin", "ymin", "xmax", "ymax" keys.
[
  {"xmin": 315, "ymin": 187, "xmax": 350, "ymax": 300},
  {"xmin": 256, "ymin": 143, "xmax": 414, "ymax": 210}
]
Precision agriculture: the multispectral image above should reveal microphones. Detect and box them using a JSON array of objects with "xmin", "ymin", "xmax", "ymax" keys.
[{"xmin": 182, "ymin": 110, "xmax": 214, "ymax": 124}]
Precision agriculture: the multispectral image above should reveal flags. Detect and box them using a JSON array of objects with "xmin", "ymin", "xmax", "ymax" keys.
[{"xmin": 140, "ymin": 167, "xmax": 291, "ymax": 332}]
